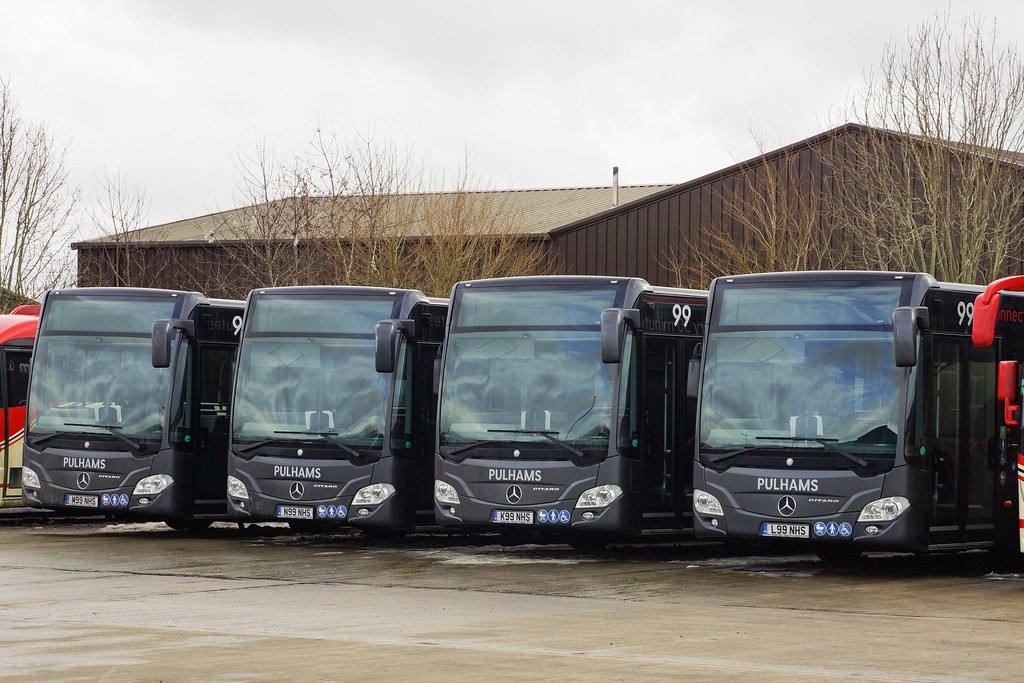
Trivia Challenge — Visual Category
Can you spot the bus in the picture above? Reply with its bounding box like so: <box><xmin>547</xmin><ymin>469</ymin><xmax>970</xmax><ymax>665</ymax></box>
<box><xmin>22</xmin><ymin>285</ymin><xmax>247</xmax><ymax>534</ymax></box>
<box><xmin>0</xmin><ymin>301</ymin><xmax>45</xmax><ymax>521</ymax></box>
<box><xmin>970</xmin><ymin>274</ymin><xmax>1024</xmax><ymax>563</ymax></box>
<box><xmin>684</xmin><ymin>268</ymin><xmax>1018</xmax><ymax>571</ymax></box>
<box><xmin>432</xmin><ymin>274</ymin><xmax>742</xmax><ymax>557</ymax></box>
<box><xmin>218</xmin><ymin>285</ymin><xmax>485</xmax><ymax>543</ymax></box>
